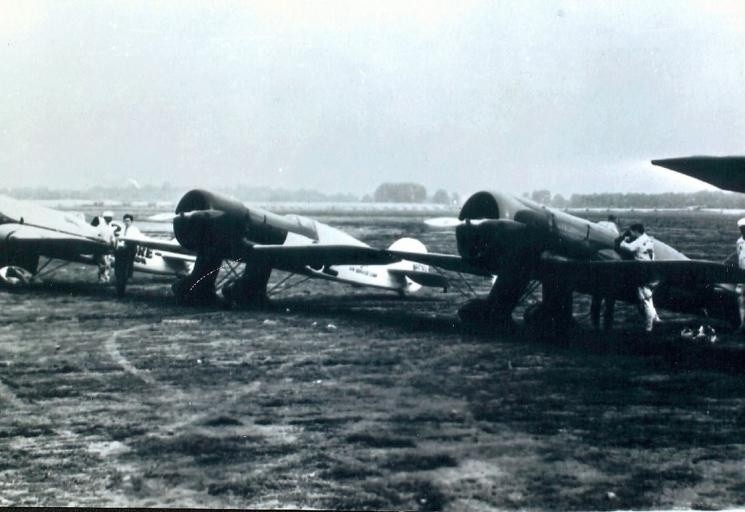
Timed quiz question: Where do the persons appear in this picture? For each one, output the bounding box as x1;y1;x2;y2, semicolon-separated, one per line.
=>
615;223;661;334
94;212;119;283
598;215;619;237
122;214;143;281
735;217;745;334
590;292;619;333
